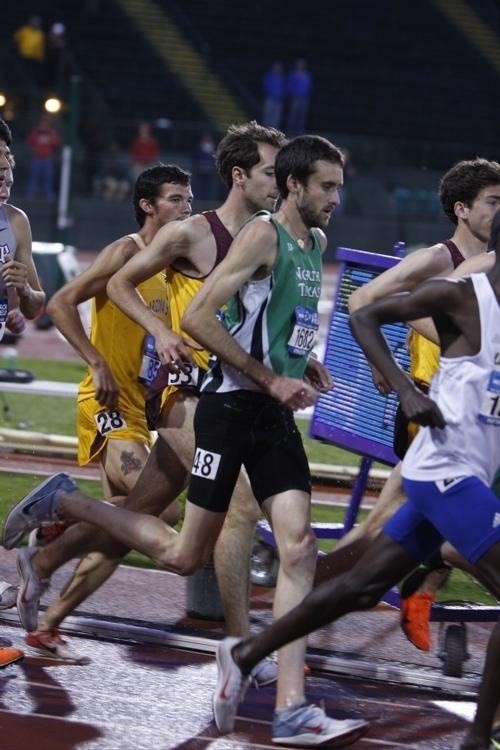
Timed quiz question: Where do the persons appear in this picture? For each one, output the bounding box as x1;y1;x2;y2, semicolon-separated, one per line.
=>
211;205;500;750
18;119;313;689
10;14;47;90
1;120;45;667
1;153;28;335
0;132;369;745
26;113;62;207
190;132;217;201
301;315;441;575
47;23;71;84
315;155;500;652
129;121;156;186
262;59;310;140
11;167;195;668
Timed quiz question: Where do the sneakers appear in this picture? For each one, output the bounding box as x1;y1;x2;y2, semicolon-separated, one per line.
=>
213;636;372;750
0;471;92;668
400;568;437;651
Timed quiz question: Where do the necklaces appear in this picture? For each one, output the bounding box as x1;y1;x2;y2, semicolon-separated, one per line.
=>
278;207;312;250
